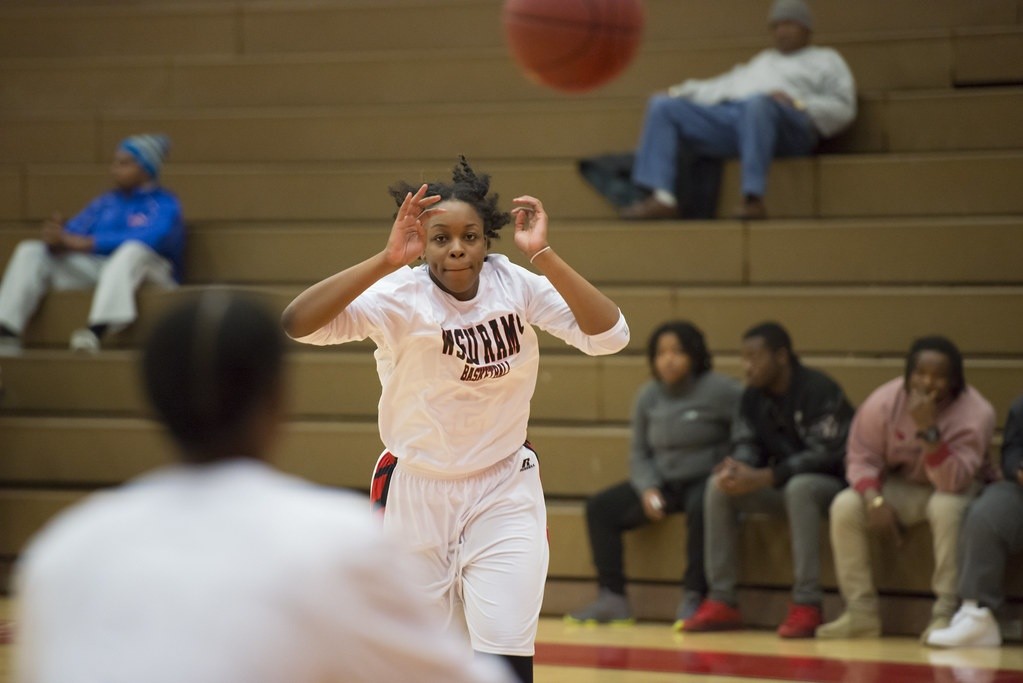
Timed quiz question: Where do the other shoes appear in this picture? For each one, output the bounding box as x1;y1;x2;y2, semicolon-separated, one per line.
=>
618;198;682;220
71;328;101;353
736;195;769;220
0;336;22;360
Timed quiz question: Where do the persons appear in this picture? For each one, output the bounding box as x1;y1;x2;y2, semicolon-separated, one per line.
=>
0;131;188;356
279;154;630;683
563;320;1023;648
623;0;858;219
12;286;512;683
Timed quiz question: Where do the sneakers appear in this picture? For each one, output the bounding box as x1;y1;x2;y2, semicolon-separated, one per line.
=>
815;604;881;639
565;588;636;630
781;607;826;639
921;605;1002;650
672;592;745;632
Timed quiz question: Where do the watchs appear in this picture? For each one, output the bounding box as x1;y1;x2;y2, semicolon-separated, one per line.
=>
918;427;940;442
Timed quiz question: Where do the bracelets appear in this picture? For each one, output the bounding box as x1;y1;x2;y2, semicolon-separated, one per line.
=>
866;496;884;508
530;246;550;264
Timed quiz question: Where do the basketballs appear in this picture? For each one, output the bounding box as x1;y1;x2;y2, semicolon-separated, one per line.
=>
504;0;643;92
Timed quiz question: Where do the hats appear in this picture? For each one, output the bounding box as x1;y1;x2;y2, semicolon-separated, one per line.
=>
767;0;816;32
122;133;170;179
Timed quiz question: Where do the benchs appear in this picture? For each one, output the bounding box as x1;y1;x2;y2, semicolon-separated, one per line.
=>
0;0;1023;593
